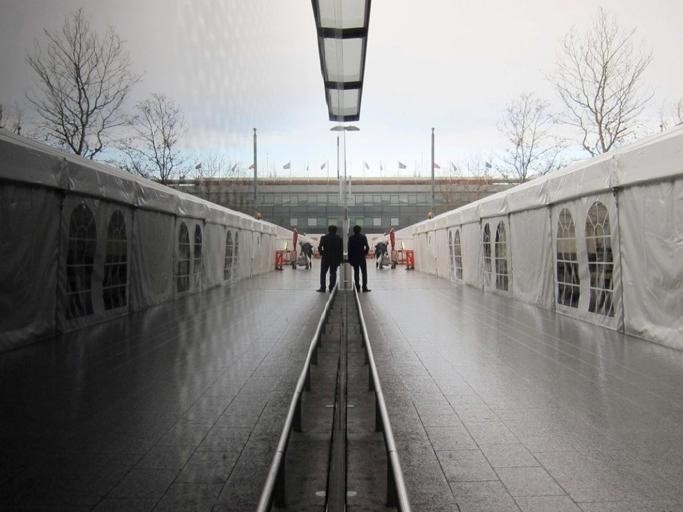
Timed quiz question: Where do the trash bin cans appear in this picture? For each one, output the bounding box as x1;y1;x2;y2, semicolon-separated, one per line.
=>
276;249;283;270
405;250;415;269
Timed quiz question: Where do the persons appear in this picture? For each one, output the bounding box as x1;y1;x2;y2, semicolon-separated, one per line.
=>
315;225;342;293
348;225;372;292
299;241;313;270
374;241;389;269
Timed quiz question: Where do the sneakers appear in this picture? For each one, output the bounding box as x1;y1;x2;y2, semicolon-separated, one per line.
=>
316;289;332;292
358;289;371;292
